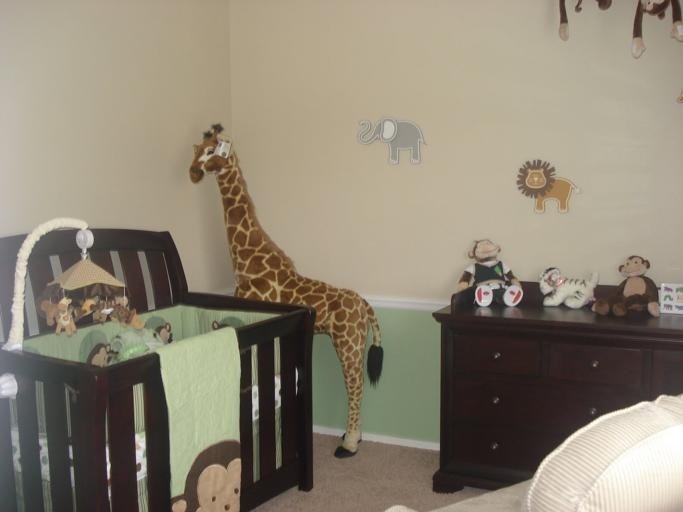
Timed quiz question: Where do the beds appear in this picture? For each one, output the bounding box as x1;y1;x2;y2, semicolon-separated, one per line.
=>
0;231;316;512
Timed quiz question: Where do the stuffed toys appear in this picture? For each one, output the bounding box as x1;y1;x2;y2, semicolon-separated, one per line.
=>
187;121;385;461
457;237;525;309
0;215;146;353
557;0;613;41
631;0;683;60
536;267;601;311
596;255;663;320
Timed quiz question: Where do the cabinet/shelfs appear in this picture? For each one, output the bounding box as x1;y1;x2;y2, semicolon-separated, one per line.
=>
431;281;683;494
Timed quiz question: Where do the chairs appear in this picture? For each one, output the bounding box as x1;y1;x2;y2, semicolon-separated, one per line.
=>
381;395;683;512
